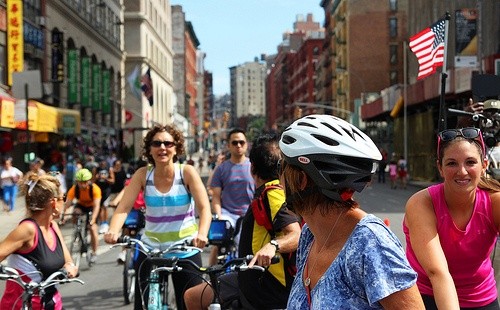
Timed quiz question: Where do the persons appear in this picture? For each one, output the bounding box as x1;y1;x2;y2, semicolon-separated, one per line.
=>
457;98;500;182
206;129;258;279
24;157;48;190
188;150;230;170
109;178;146;264
105;125;213;310
278;114;426;310
0;158;23;211
378;147;408;190
60;168;102;266
0;174;79;310
403;127;500;310
49;154;147;234
184;136;302;310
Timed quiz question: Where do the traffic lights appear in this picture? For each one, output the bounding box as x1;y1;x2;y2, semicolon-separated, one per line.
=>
295;106;303;119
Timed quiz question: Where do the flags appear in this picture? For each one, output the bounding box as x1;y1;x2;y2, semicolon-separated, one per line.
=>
143;69;154;106
127;64;142;102
409;18;445;81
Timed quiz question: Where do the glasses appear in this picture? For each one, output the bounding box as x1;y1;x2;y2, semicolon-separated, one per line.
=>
150;140;177;149
231;139;246;146
436;127;485;161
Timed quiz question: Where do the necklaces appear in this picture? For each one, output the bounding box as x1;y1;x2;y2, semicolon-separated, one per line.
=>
304;207;343;286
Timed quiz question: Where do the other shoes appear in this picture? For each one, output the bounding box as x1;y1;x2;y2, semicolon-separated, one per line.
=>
99;224;108;233
118;256;126;263
90;256;98;263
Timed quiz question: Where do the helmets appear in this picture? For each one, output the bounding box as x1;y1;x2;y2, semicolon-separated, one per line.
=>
74;168;92;182
278;114;383;204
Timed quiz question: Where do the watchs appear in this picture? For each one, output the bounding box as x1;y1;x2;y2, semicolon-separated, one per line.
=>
269;240;280;253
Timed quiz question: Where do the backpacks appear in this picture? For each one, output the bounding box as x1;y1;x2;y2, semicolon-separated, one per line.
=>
250;184;306;279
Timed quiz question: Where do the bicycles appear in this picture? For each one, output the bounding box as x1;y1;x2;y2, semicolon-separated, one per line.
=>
148;238;280;310
59;210;93;271
107;203;147;305
0;264;85;310
108;234;210;310
